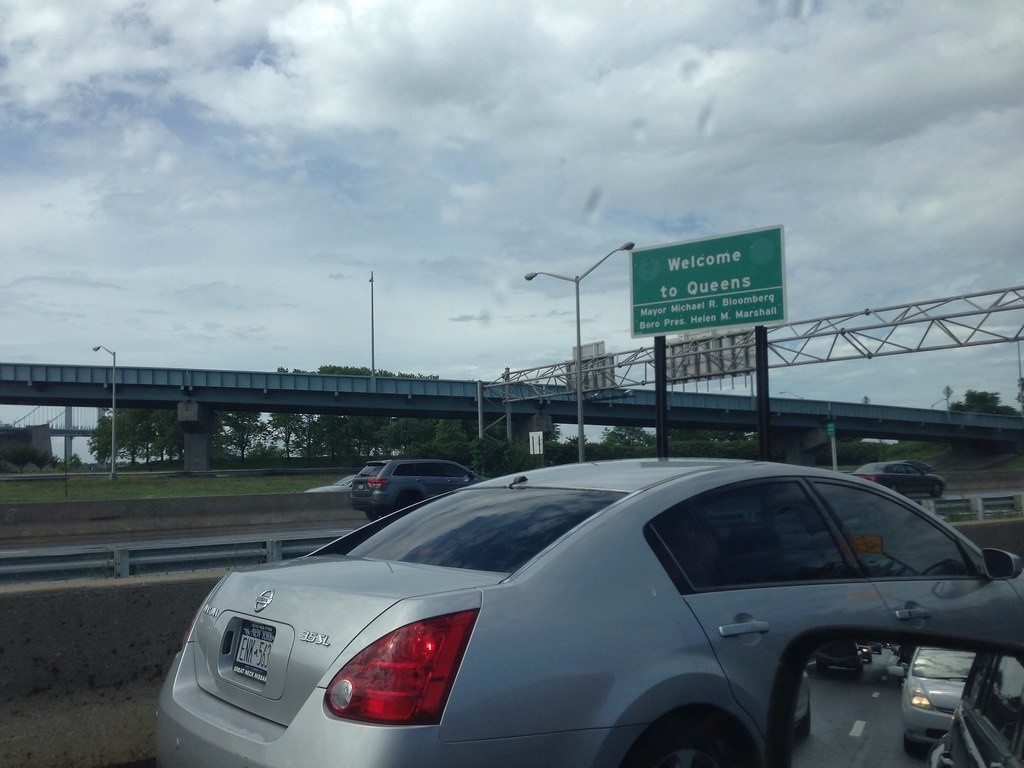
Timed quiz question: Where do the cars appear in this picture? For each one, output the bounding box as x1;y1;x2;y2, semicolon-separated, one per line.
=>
791;668;812;736
304;474;356;493
845;460;947;498
895;647;976;757
813;638;917;674
895;458;936;472
146;457;1024;768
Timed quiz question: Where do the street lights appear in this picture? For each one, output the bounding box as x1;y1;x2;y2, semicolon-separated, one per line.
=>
522;239;638;465
930;398;948;409
367;271;376;376
91;344;119;480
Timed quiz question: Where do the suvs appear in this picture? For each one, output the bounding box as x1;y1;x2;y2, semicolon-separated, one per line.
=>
349;459;492;524
